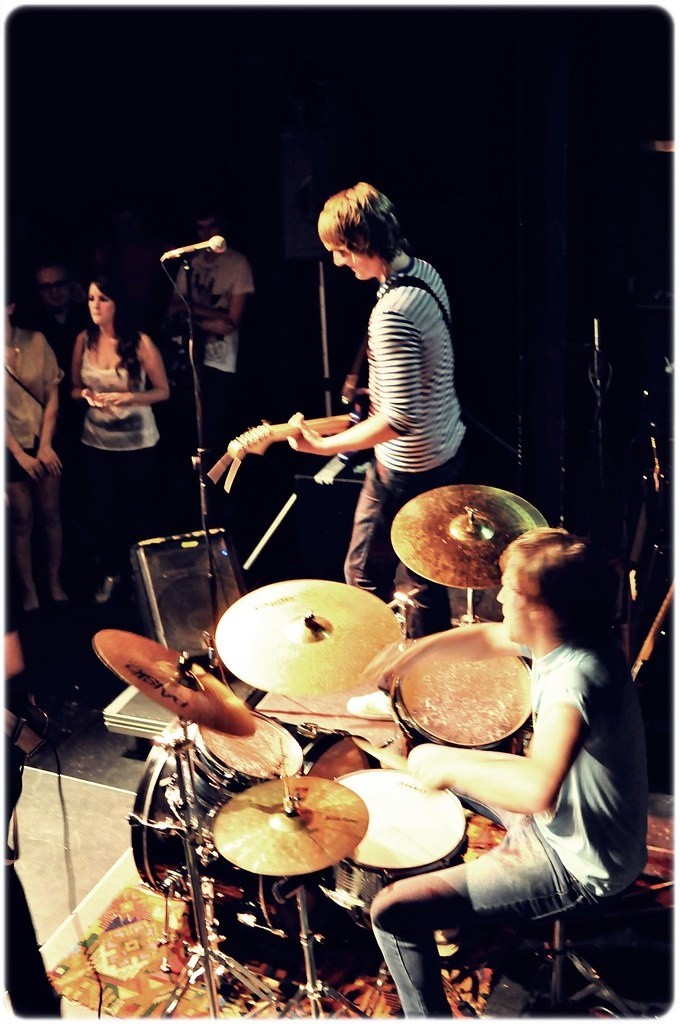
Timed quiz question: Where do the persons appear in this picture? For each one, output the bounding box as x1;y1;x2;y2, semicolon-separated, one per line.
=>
5;214;256;616
366;527;649;1016
285;181;467;605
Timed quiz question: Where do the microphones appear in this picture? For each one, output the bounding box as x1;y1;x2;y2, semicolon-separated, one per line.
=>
159;236;227;261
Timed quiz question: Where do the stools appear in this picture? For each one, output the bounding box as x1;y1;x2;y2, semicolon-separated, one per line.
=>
502;885;637;1019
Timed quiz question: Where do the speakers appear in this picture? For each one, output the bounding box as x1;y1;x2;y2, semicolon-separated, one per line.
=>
130;528;249;658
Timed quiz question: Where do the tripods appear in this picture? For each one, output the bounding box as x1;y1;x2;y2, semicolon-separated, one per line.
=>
160;713;369;1019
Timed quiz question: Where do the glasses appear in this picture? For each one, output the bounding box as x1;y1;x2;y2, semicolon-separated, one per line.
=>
38;279;68;291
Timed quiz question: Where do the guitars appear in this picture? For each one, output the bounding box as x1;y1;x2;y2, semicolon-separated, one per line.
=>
227;388;373;467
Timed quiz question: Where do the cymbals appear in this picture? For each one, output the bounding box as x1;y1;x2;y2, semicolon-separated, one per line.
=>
212;776;369;876
214;578;402;697
390;484;551;591
91;629;256;739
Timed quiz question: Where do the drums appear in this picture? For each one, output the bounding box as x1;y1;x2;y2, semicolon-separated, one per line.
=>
391;655;533;752
130;710;468;918
